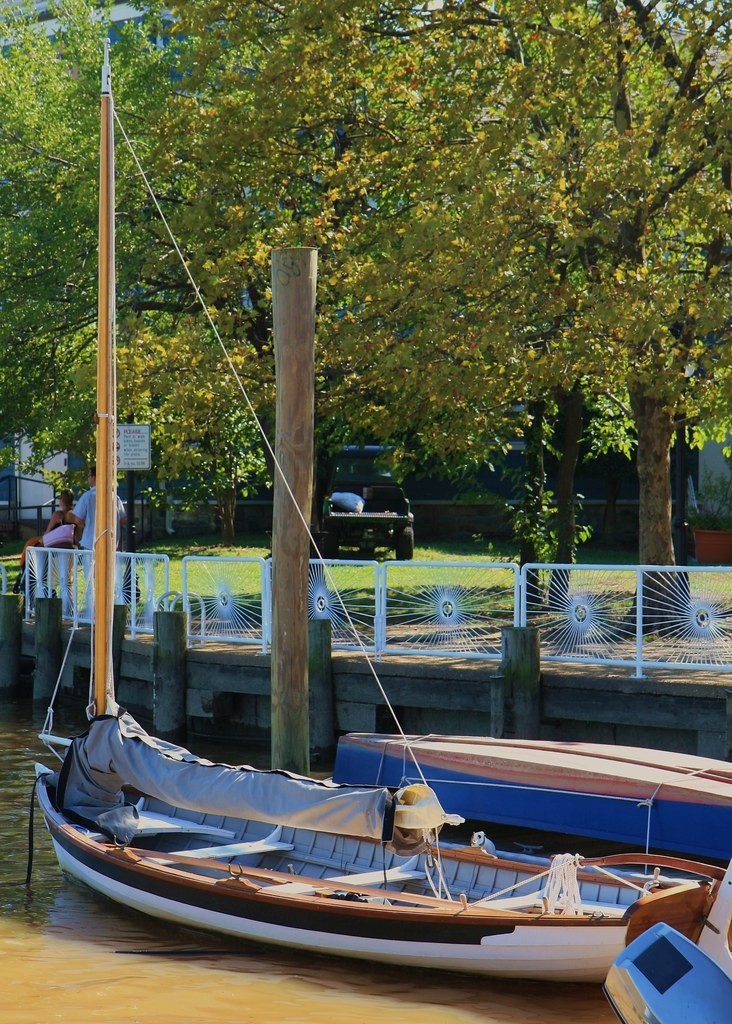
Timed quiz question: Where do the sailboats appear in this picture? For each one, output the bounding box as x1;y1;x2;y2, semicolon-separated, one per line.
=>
17;34;723;985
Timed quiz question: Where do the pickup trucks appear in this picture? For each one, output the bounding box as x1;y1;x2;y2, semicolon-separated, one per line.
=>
314;444;416;561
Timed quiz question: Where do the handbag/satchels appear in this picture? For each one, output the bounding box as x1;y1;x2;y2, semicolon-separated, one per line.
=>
42;518;75;548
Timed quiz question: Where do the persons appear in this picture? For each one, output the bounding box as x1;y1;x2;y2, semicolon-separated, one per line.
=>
72;467;127;620
45;489;85;550
